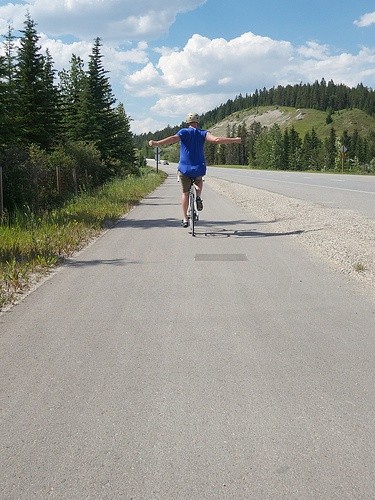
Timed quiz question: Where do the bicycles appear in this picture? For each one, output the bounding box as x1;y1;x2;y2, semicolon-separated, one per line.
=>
176;172;206;237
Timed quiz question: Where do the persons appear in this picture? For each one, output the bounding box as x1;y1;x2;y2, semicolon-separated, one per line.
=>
148;112;242;228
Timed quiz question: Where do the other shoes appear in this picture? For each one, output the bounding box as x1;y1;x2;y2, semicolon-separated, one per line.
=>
196;196;203;211
181;219;188;227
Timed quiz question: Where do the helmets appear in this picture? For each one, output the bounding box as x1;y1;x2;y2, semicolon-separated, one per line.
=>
186;112;200;124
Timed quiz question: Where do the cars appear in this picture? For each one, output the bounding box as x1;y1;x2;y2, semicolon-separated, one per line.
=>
156;159;169;165
